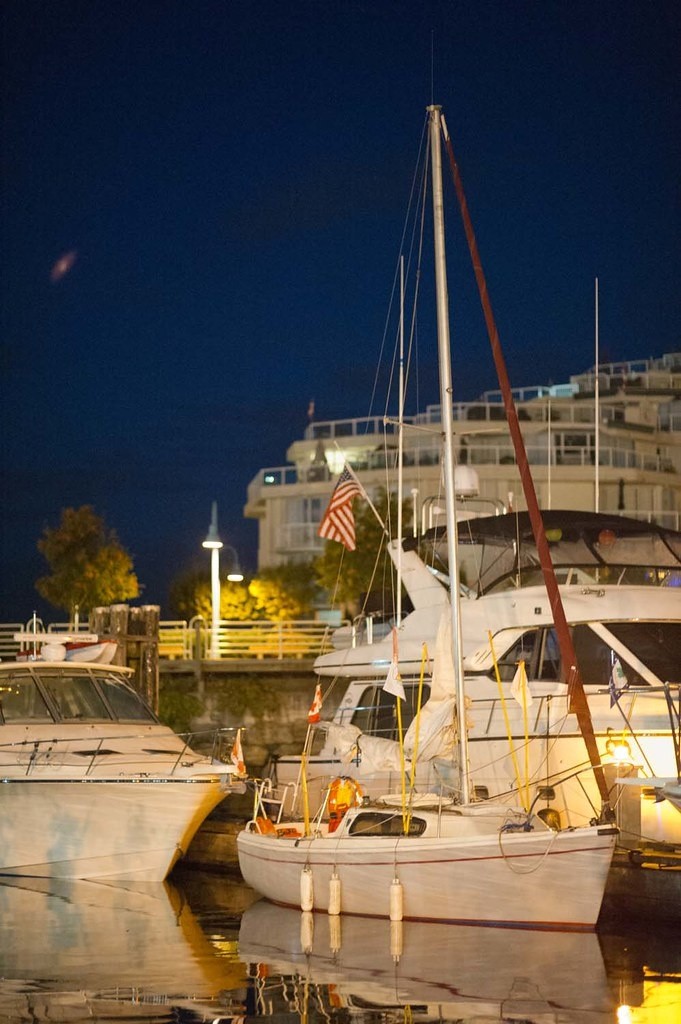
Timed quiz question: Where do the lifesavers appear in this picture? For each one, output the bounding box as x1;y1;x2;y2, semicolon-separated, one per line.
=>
326;775;365;820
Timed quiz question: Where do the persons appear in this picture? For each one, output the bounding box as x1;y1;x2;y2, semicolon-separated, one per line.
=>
327;776;366;831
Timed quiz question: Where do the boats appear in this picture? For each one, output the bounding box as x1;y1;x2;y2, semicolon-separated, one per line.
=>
0;632;240;883
258;507;681;829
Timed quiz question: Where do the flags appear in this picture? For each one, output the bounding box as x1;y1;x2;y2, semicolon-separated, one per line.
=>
387;660;407;703
308;685;323;723
511;663;534;708
230;729;246;775
319;467;367;551
610;655;629;708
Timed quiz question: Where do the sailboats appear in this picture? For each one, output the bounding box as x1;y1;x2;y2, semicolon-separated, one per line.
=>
236;101;619;931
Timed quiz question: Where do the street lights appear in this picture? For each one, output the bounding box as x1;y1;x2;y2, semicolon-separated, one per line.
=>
201;501;223;658
